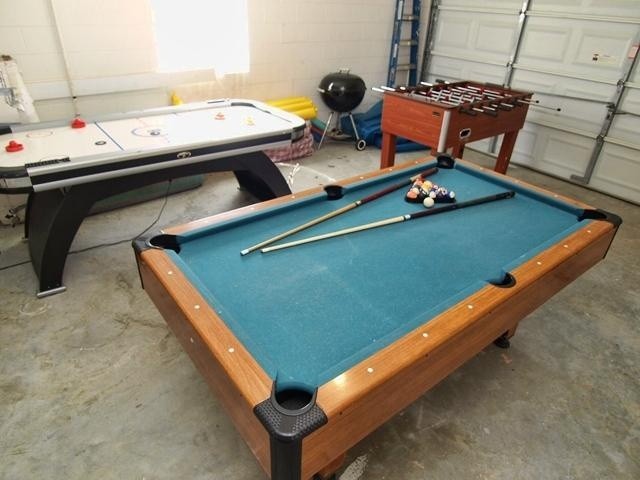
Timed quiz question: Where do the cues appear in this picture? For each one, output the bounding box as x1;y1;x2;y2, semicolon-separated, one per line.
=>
260;190;515;252
238;166;439;256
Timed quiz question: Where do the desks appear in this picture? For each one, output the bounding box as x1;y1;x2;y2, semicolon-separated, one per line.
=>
372;78;534;176
1;99;307;300
134;152;621;476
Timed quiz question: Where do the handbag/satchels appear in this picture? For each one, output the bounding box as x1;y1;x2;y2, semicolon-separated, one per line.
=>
262;121;314;164
